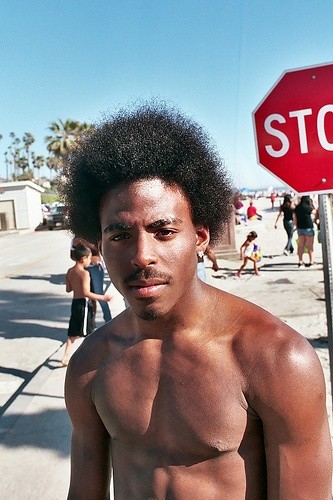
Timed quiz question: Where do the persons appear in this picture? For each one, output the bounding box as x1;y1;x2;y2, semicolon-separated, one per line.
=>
275;194;296;256
270;193;275;210
72;236;112;336
314;208;320;229
65;109;333;500
246;201;262;220
62;243;112;366
294;195;315;267
197;248;218;282
237;231;260;277
234;192;248;226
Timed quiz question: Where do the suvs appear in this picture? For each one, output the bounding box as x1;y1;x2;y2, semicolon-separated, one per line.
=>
46;206;71;230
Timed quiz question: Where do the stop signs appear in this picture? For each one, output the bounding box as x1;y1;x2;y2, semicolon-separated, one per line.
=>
251;61;333;195
